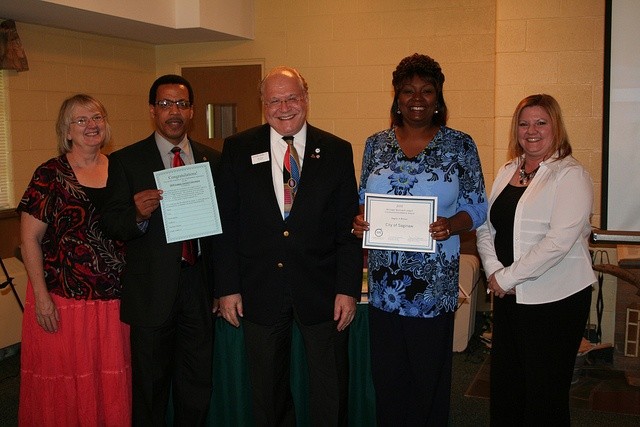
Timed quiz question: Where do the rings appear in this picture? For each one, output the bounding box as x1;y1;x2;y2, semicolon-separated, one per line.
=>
351;229;355;234
446;228;451;236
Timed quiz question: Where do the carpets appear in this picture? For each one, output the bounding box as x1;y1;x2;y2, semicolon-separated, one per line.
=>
463;344;639;416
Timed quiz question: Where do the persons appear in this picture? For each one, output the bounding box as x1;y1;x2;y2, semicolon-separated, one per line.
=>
349;52;487;427
475;96;599;427
16;93;135;427
213;65;359;427
101;73;223;426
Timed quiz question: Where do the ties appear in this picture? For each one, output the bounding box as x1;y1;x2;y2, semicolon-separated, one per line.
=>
282;136;301;220
171;147;198;267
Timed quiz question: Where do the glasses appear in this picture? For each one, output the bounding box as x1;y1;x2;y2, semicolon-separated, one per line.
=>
264;98;306;106
69;115;104;127
156;100;191;109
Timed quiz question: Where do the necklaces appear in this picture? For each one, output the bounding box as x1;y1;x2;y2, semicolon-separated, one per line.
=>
519;160;541;185
